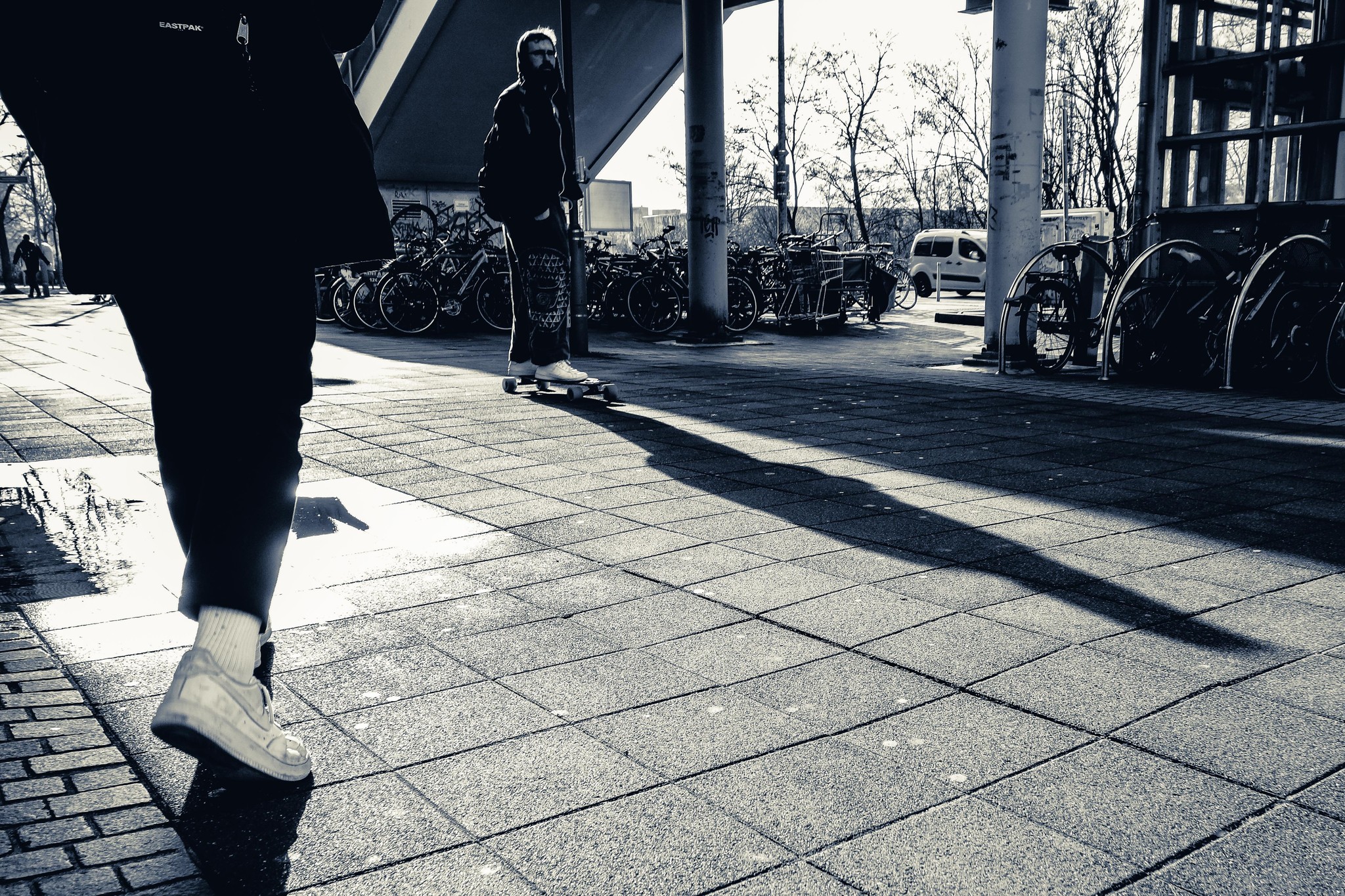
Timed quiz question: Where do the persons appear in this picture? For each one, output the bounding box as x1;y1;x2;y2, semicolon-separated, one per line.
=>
0;0;378;784
13;234;52;297
38;237;54;294
486;31;590;383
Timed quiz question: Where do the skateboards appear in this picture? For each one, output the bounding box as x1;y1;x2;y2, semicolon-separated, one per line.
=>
501;375;621;401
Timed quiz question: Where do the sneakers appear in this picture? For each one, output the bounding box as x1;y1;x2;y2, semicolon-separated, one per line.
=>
508;360;536;376
535;360;588;381
150;648;312;781
255;612;271;668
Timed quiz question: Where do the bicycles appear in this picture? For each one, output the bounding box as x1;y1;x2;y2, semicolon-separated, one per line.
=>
1004;210;1345;401
314;196;918;339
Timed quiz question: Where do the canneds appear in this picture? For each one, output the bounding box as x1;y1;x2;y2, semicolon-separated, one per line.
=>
575;156;586;182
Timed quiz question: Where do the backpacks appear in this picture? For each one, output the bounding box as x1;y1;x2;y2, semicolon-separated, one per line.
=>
478;126;505;223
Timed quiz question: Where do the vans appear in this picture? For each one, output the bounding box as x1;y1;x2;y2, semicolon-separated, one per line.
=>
908;229;987;298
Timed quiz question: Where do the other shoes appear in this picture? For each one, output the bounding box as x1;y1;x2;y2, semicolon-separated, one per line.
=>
89;295;101;302
27;293;34;297
35;294;42;298
